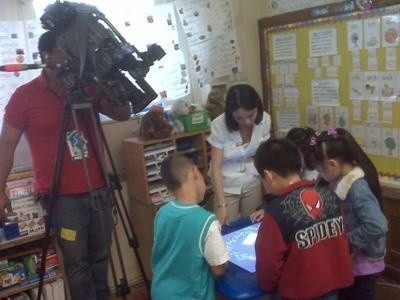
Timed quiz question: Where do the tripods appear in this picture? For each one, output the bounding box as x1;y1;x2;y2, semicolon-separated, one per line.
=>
36;89;150;300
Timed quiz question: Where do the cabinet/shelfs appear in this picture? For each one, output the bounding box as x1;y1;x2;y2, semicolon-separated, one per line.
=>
123;127;213;282
0;169;72;300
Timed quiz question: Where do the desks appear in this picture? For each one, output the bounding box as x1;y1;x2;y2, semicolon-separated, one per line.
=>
214;215;273;300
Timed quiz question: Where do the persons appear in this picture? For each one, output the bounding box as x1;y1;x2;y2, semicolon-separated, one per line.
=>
249;127;388;300
0;31;131;300
207;84;272;226
150;156;229;300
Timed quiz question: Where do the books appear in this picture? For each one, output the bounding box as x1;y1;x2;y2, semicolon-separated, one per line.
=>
0;179;66;300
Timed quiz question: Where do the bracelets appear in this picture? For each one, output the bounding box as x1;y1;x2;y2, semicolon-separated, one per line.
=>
216;203;227;208
